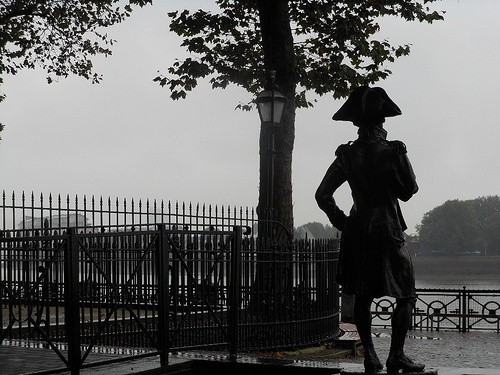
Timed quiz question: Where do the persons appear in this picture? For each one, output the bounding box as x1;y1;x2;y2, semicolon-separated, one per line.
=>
314;87;426;374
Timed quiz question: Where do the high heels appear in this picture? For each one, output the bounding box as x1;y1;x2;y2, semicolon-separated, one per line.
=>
362;360;384;374
387;357;425;373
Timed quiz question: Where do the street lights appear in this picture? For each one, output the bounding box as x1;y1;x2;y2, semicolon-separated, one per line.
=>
255;68;287;310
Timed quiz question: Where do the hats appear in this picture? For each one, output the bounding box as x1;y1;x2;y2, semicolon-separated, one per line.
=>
332;87;402;120
